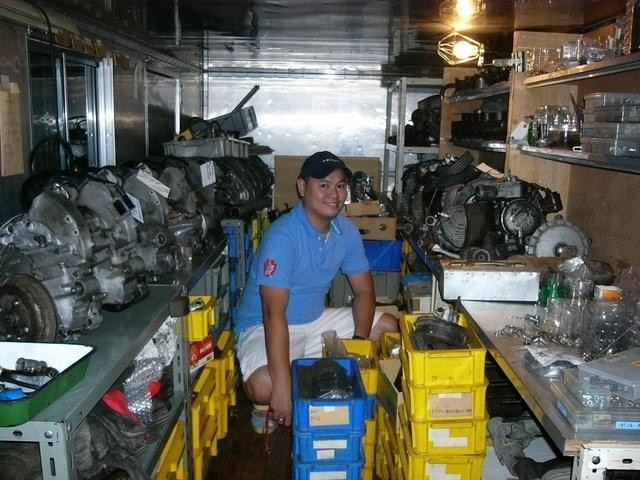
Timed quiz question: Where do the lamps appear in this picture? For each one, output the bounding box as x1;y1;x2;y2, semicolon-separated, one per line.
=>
437;34;483;64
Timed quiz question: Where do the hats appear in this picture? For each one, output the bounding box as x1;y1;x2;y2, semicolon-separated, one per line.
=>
298;150;354;183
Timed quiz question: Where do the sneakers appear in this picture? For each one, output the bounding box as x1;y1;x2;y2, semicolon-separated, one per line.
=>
250;403;280;435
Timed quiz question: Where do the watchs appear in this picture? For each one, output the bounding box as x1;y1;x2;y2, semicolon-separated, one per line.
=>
353;335;369;340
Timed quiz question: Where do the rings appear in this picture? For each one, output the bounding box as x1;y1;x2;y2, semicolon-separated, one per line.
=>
279;417;285;421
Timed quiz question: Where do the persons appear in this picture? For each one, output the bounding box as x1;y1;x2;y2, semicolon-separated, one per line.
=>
235;152;399;434
